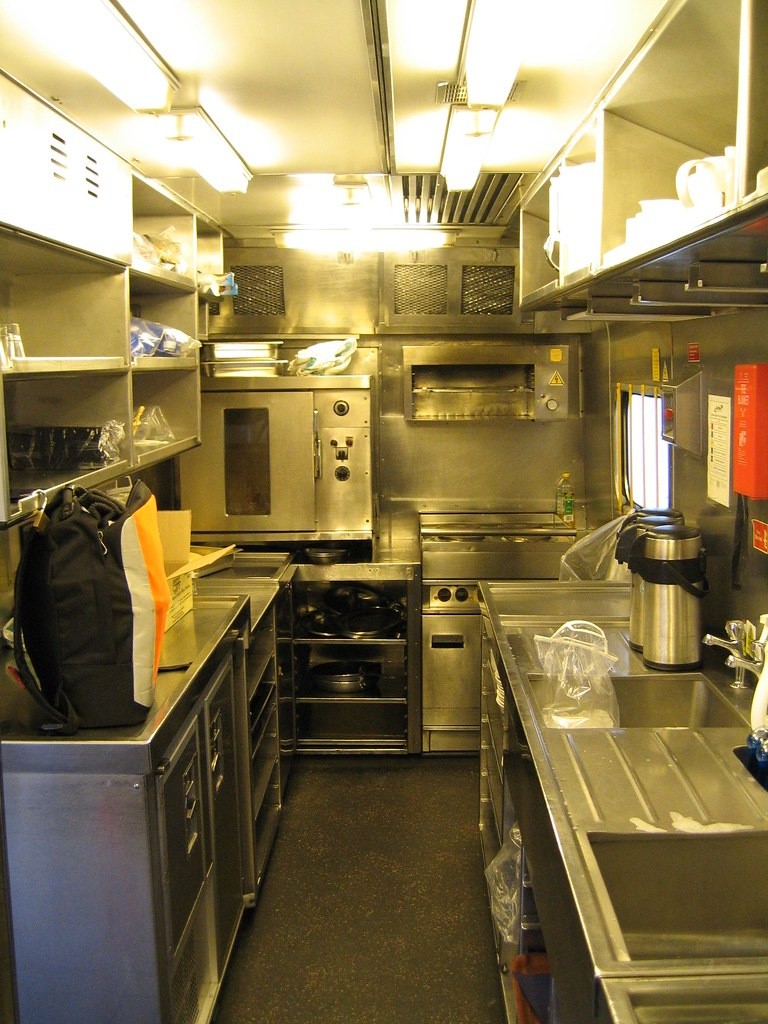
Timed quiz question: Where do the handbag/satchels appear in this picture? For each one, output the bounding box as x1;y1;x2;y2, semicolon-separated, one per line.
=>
7;478;172;735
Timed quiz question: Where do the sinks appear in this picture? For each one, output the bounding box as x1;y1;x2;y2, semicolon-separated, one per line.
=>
526;672;749;729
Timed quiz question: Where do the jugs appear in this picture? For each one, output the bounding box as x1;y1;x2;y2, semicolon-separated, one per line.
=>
615;507;711;672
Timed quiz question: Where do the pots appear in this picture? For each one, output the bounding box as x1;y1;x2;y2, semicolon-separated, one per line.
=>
310;660;382;692
303;546;353;563
295;584;398;639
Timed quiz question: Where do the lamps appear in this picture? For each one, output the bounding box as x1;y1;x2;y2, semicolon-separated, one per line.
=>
1;0;549;194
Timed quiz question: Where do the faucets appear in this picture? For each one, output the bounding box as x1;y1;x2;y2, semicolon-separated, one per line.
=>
726;641;765;678
701;619;749;690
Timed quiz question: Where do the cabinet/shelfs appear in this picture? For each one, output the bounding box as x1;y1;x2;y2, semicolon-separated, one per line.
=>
479;616;559;1024
156;540;421;1024
1;69;224;531
520;0;767;316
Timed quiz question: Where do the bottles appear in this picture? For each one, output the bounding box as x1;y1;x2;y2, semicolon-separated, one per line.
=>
556;472;575;526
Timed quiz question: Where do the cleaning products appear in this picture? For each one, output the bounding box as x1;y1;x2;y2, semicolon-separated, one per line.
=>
750;612;767;734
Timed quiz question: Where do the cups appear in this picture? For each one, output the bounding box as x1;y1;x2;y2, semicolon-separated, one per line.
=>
0;323;25;356
